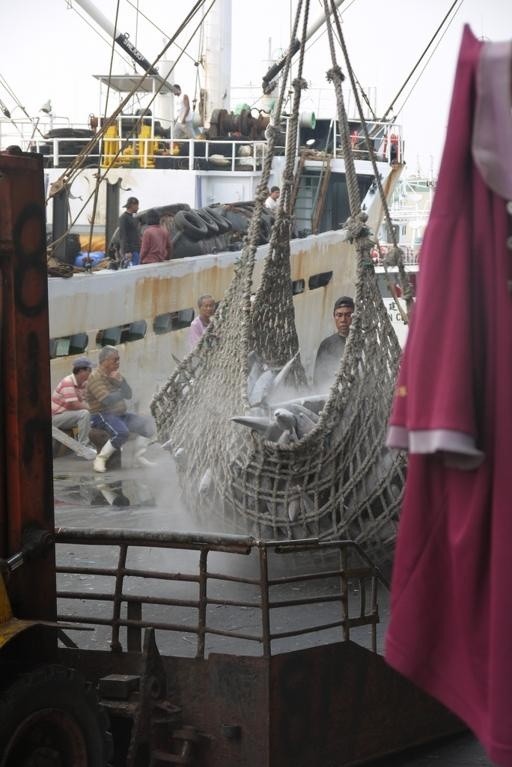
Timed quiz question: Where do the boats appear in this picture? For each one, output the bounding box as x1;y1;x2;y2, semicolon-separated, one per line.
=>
363;141;447;352
0;0;416;506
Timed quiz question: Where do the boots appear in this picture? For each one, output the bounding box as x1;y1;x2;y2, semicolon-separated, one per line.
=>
93;441;117;473
130;434;157;469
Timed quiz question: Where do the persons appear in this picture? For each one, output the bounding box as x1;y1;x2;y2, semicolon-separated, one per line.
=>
162;84;196;155
87;344;154;473
313;296;354;393
264;186;286;211
51;357;97;456
119;197;141;268
139;208;172;263
191;295;216;347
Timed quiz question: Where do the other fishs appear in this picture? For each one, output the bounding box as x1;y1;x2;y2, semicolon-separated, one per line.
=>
146;349;409;577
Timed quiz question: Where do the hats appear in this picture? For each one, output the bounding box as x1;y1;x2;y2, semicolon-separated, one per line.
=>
122;196;140;208
333;296;355;309
72;357;99;369
